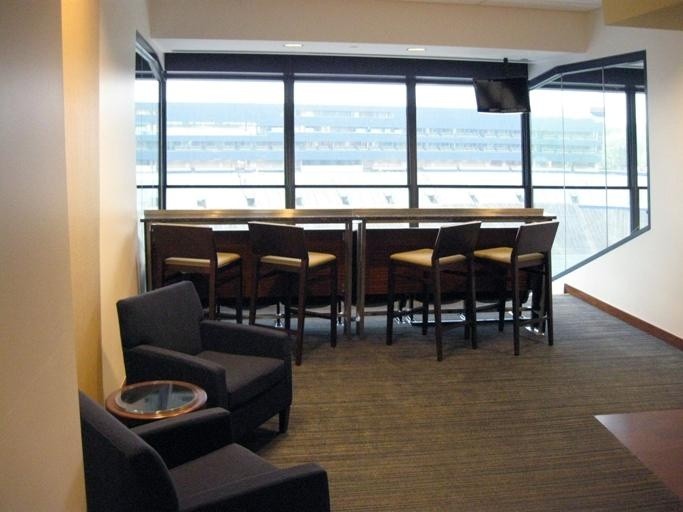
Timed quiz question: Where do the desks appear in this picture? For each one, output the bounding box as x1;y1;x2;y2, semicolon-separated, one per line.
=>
103;378;209;422
138;217;358;342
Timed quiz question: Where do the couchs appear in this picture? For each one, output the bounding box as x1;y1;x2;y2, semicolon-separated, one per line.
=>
116;279;297;444
77;386;329;512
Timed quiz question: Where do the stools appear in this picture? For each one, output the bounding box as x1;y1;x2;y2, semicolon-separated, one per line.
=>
384;220;484;361
473;218;561;356
152;222;245;326
247;219;340;370
